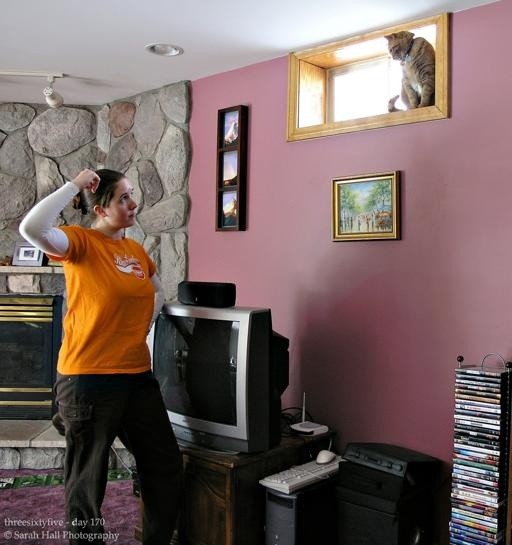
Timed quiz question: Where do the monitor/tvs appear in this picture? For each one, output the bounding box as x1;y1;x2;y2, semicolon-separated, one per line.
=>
146;302;289;458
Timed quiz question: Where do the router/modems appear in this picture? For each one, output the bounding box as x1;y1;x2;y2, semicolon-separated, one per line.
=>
289;392;329;436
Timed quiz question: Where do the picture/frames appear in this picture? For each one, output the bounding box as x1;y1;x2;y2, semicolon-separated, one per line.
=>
214;104;250;231
12;242;46;267
331;169;402;242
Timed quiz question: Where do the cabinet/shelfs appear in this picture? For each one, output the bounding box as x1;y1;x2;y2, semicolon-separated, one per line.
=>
131;413;338;545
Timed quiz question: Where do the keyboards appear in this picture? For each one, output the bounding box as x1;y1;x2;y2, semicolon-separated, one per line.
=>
258;453;348;495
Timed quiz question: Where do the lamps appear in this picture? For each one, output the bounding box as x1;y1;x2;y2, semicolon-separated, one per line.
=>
42;75;64;109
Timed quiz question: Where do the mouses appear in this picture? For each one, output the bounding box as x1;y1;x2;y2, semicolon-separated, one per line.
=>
315;450;336;464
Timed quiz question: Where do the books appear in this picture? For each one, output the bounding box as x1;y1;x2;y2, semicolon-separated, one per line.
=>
448;363;511;545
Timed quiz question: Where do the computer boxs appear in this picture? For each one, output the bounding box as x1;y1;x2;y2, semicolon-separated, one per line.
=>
265;476;337;545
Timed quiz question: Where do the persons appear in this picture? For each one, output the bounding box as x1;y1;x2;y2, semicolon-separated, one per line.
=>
17;167;189;545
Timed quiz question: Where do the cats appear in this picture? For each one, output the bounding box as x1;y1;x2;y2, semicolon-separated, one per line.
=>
384;31;435;112
